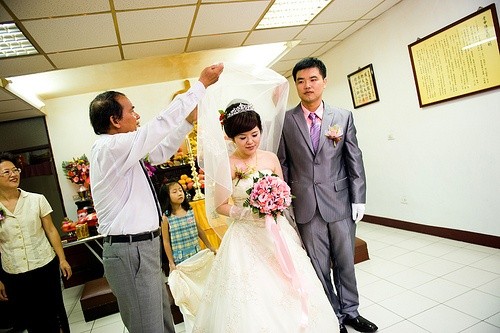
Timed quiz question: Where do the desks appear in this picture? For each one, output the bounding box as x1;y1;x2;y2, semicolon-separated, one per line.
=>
189;199;228;256
63;234;103;264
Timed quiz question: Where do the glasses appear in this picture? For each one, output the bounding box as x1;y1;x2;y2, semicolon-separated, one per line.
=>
0;168;21;176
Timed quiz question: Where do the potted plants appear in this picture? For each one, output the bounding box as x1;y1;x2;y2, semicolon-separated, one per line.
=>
62;154;96;216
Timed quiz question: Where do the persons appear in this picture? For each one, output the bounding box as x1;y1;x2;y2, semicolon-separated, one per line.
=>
161;180;217;273
89;61;225;333
0;153;72;333
169;98;340;333
261;56;378;333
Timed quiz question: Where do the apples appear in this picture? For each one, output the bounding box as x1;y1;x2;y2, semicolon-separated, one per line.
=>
177;174;194;190
197;169;205;183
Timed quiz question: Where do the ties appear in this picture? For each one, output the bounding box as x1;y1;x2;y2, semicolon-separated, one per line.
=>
309;113;320;153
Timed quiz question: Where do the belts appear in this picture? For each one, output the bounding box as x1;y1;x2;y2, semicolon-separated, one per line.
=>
104;227;161;243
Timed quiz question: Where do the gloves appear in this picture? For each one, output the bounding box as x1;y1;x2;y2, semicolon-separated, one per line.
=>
229;206;264;221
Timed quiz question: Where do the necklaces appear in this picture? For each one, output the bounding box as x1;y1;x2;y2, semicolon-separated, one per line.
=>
239;153;258;168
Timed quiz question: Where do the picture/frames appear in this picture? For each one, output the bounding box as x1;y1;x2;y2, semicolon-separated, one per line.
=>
347;63;380;109
408;3;500;108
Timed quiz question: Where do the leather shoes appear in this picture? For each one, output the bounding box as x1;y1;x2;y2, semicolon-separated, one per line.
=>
340;324;348;333
342;315;378;333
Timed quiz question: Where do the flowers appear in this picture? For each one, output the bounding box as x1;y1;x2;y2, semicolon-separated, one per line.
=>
143;152;156;177
324;124;343;147
234;164;296;225
